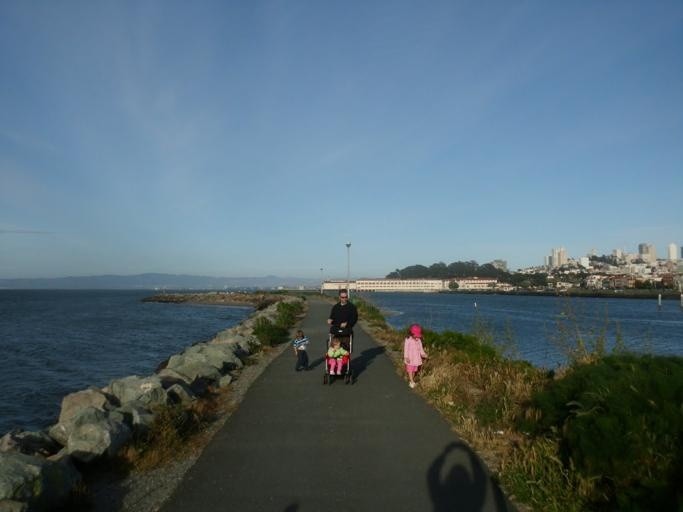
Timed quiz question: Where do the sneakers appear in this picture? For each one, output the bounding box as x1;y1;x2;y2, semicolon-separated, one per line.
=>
295;366;311;372
329;371;342;375
409;382;416;388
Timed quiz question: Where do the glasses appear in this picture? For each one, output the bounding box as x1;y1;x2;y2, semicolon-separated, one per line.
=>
340;296;348;299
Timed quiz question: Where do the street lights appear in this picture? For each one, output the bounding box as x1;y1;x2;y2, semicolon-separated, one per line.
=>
320;268;324;282
345;241;351;298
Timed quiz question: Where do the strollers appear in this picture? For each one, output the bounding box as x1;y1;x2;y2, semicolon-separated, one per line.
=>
322;325;356;386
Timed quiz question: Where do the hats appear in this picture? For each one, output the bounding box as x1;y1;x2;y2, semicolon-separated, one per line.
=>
409;324;422;338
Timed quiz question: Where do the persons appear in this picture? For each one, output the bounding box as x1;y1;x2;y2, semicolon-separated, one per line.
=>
326;335;349;375
402;324;429;388
293;330;312;370
327;289;358;334
